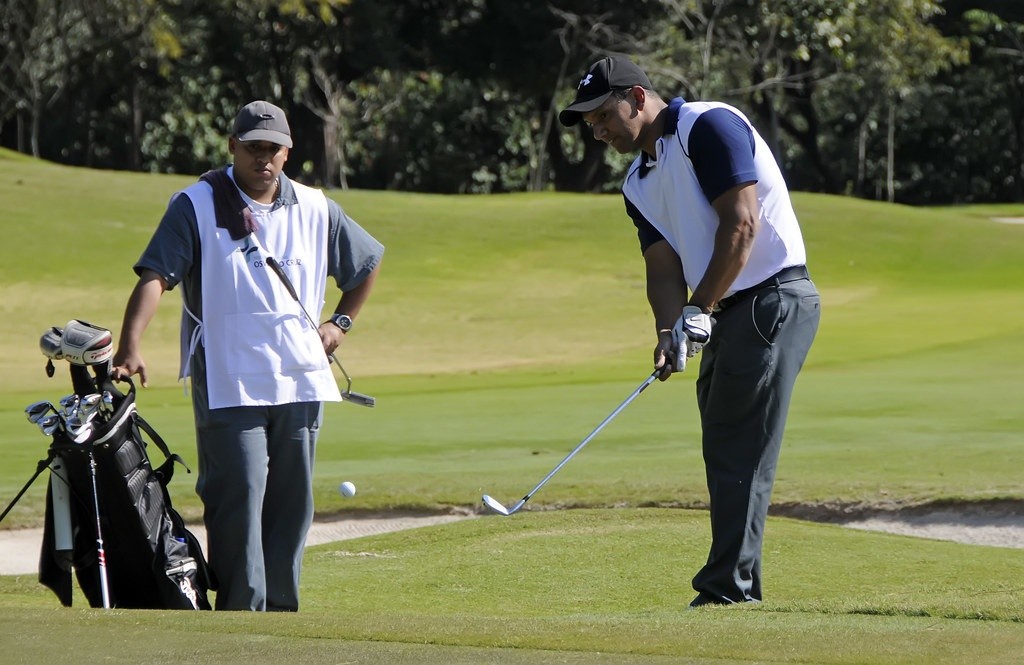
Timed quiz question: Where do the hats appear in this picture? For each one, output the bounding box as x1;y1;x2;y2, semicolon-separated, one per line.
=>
232;102;293;148
559;56;652;127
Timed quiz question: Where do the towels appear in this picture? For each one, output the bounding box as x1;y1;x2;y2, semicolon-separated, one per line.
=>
198;166;260;241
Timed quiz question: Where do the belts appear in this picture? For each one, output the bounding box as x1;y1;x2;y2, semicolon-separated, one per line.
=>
713;265;809;312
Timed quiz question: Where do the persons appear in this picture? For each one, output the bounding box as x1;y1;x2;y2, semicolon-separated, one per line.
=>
581;54;822;604
103;100;387;611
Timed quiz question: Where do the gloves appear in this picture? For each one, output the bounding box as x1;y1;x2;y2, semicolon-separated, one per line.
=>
672;306;712;372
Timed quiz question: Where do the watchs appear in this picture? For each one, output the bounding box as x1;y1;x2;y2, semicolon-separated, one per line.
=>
329;313;353;334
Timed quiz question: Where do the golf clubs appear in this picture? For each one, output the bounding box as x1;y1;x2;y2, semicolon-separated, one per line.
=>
482;369;664;517
24;391;114;446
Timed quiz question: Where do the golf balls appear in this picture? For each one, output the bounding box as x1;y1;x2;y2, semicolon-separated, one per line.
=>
340;481;357;498
